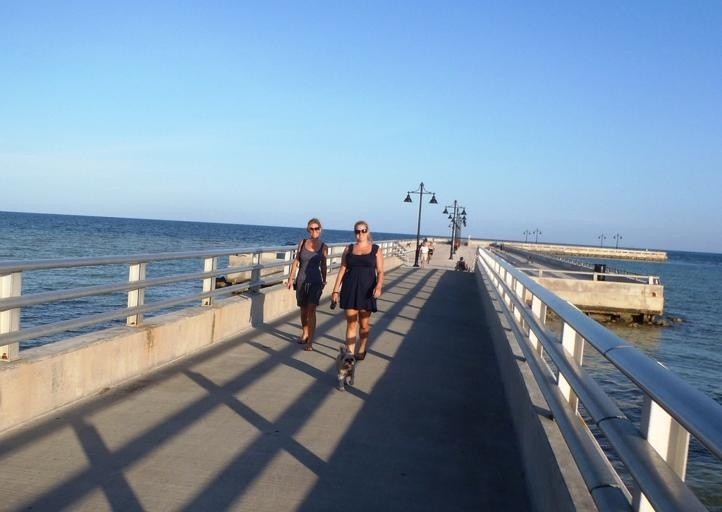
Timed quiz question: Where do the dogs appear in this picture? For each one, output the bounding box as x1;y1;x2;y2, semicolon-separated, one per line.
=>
335;345;357;391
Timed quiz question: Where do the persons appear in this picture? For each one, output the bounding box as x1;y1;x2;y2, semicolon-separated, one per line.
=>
288;218;328;351
419;242;428;268
331;221;384;360
455;257;467;271
428;242;435;264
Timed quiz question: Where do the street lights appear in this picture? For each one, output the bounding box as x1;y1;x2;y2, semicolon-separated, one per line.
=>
533;228;542;244
597;232;606;246
403;181;439;267
613;232;623;248
443;200;467;260
523;229;531;242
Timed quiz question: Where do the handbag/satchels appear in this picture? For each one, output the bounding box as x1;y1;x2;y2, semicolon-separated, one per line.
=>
292;281;297;291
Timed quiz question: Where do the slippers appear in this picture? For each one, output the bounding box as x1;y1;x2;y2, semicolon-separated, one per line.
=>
356;351;366;361
298;336;313;351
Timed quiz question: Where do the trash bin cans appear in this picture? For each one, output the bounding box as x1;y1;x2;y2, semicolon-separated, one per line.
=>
593;264;606;280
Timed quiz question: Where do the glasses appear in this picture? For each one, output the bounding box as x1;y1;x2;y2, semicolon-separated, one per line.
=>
354;230;368;234
310;228;320;231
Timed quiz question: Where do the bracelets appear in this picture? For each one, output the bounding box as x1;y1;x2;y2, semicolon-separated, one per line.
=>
333;292;338;293
